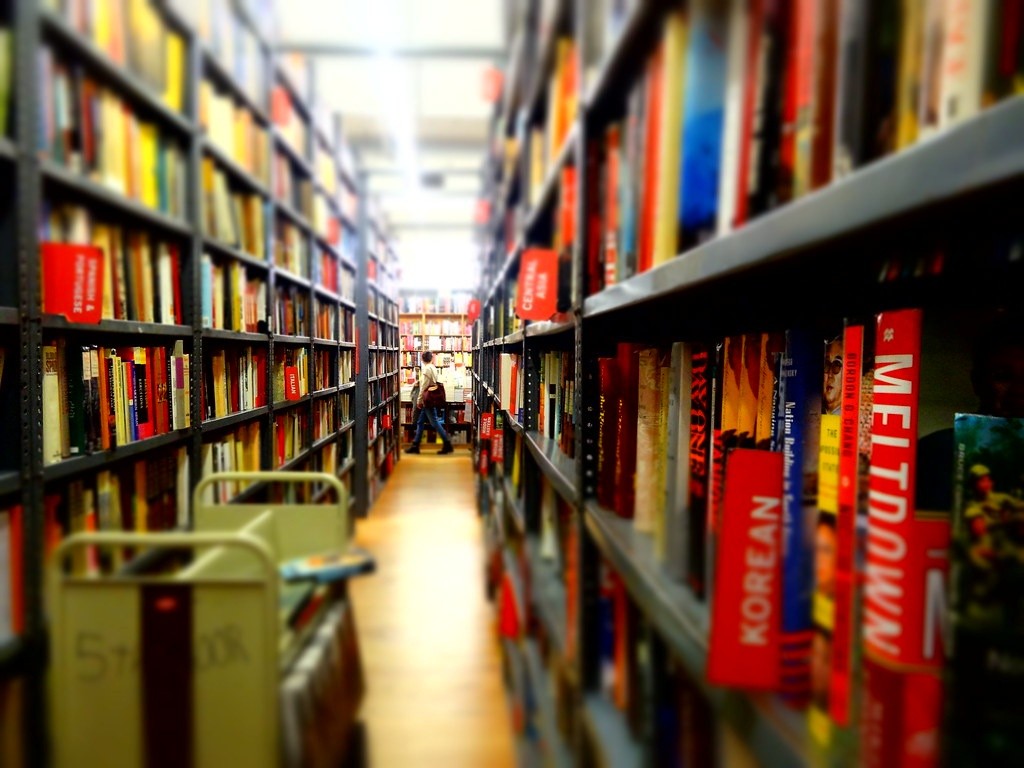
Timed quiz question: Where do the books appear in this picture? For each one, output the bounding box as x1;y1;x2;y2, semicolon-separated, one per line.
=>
471;0;1024;768
0;0;398;768
399;290;473;445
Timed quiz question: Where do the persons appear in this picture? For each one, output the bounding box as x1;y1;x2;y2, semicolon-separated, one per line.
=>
404;351;454;455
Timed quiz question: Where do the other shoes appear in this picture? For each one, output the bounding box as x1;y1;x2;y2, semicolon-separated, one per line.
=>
436;446;452;455
405;446;419;453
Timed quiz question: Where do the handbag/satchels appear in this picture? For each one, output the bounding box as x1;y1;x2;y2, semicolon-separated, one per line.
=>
422;366;445;408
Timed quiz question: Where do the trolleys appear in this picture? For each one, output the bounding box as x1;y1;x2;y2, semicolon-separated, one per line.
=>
43;473;370;768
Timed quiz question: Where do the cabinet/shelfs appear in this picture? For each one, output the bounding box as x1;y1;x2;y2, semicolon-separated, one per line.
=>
465;0;1023;768
398;312;472;448
1;0;401;768
